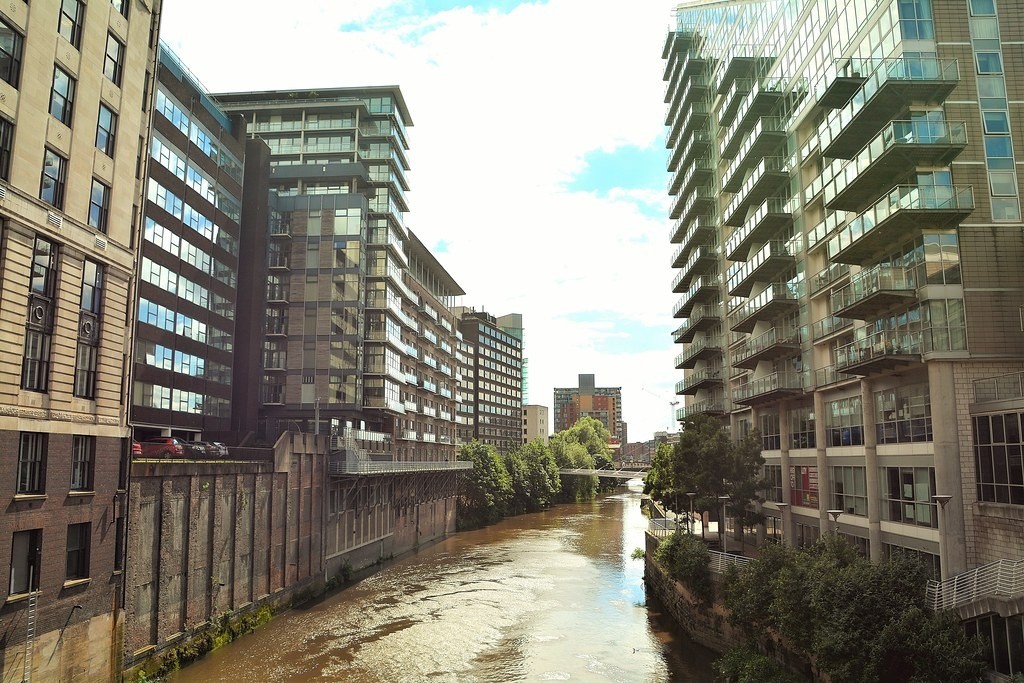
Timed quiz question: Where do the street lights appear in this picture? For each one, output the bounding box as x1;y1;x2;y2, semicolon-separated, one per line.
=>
826;508;845;547
774;502;791;564
686;492;697;542
931;494;955;607
718;496;731;574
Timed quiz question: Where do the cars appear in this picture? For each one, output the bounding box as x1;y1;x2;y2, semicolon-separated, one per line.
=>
133;439;142;460
138;436;230;460
